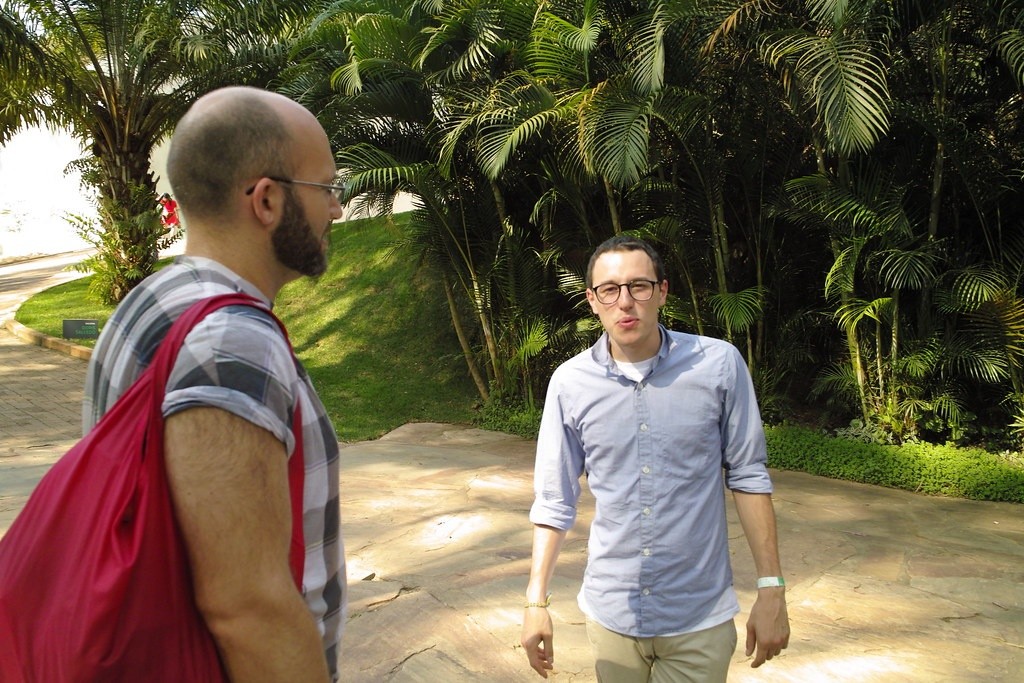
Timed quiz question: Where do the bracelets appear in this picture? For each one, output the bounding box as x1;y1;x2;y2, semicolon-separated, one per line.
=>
524;592;552;607
757;576;786;589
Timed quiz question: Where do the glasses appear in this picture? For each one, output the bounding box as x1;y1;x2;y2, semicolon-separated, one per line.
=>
592;279;661;304
246;176;346;205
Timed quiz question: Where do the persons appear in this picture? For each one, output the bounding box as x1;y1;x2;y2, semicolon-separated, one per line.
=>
80;87;347;683
160;193;181;240
521;235;790;683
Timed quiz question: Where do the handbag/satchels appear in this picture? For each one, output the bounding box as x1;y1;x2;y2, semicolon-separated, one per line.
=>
0;294;306;683
